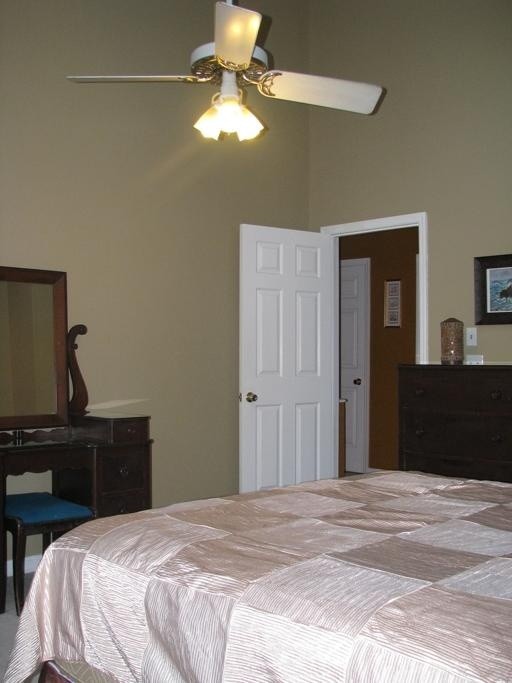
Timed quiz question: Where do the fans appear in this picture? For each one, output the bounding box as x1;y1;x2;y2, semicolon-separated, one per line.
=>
66;0;384;115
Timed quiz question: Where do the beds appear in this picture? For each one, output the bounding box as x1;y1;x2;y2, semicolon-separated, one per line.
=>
46;470;512;683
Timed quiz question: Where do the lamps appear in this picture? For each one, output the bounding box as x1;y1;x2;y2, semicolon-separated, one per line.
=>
192;72;264;141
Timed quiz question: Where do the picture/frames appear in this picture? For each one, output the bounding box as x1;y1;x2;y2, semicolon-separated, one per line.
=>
474;254;512;325
384;279;403;329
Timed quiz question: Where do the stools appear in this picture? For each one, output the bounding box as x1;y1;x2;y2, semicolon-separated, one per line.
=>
6;492;95;617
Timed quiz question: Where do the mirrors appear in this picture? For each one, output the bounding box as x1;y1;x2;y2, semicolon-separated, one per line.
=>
0;266;69;431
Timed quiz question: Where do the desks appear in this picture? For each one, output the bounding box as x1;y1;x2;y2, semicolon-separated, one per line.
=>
0;409;154;614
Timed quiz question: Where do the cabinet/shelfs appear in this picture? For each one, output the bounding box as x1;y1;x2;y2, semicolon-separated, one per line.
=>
396;364;512;484
